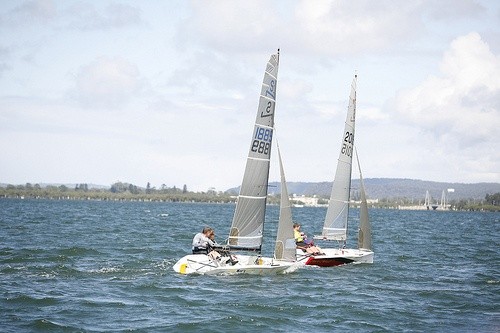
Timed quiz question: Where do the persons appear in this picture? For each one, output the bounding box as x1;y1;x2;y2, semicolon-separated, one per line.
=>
207;233;217;244
293;223;323;255
192;227;239;266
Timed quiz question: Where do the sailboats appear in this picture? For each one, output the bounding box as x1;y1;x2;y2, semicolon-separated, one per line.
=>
173;47;300;278
294;71;375;267
